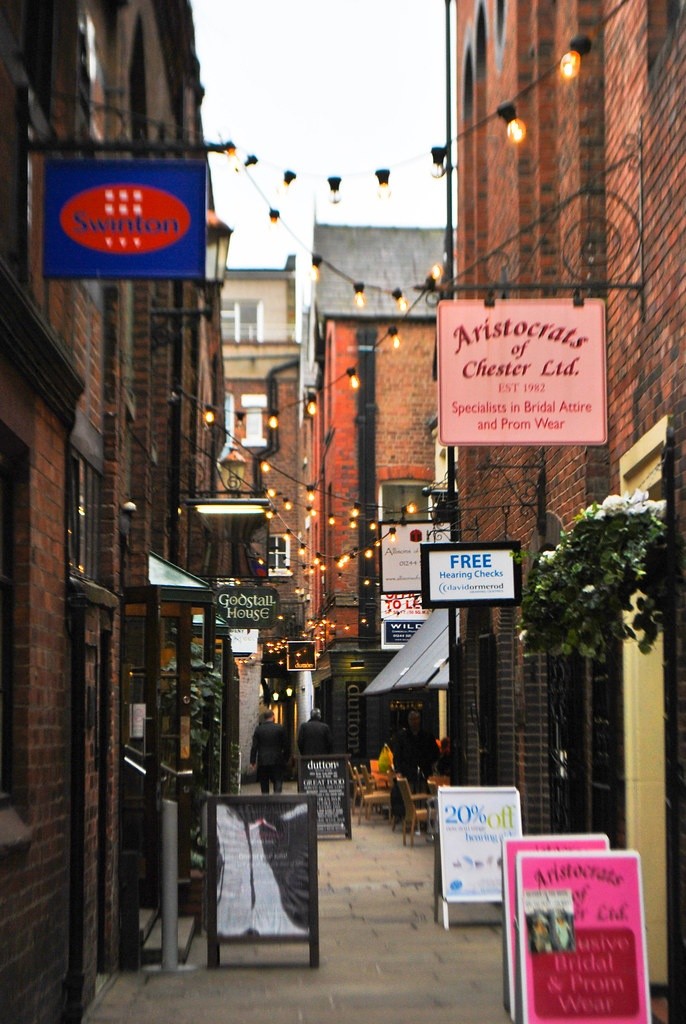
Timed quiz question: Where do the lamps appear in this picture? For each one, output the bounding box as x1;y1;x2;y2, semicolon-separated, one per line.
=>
180;442;269;499
272;691;280;700
286;686;294;697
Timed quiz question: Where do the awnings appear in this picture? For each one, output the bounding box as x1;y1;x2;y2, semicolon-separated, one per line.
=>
362;608;459;695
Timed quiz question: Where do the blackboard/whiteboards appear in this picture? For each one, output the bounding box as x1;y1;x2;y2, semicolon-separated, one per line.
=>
296;753;354;835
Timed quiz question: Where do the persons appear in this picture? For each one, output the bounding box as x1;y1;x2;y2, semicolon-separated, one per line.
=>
297;709;332;757
391;713;450;830
250;711;290;795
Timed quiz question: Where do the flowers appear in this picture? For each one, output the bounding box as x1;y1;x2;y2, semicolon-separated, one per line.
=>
509;488;670;663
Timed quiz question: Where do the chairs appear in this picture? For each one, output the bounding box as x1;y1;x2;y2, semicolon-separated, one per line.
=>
396;774;437;848
354;767;392;827
347;762;378;815
360;765;390;820
